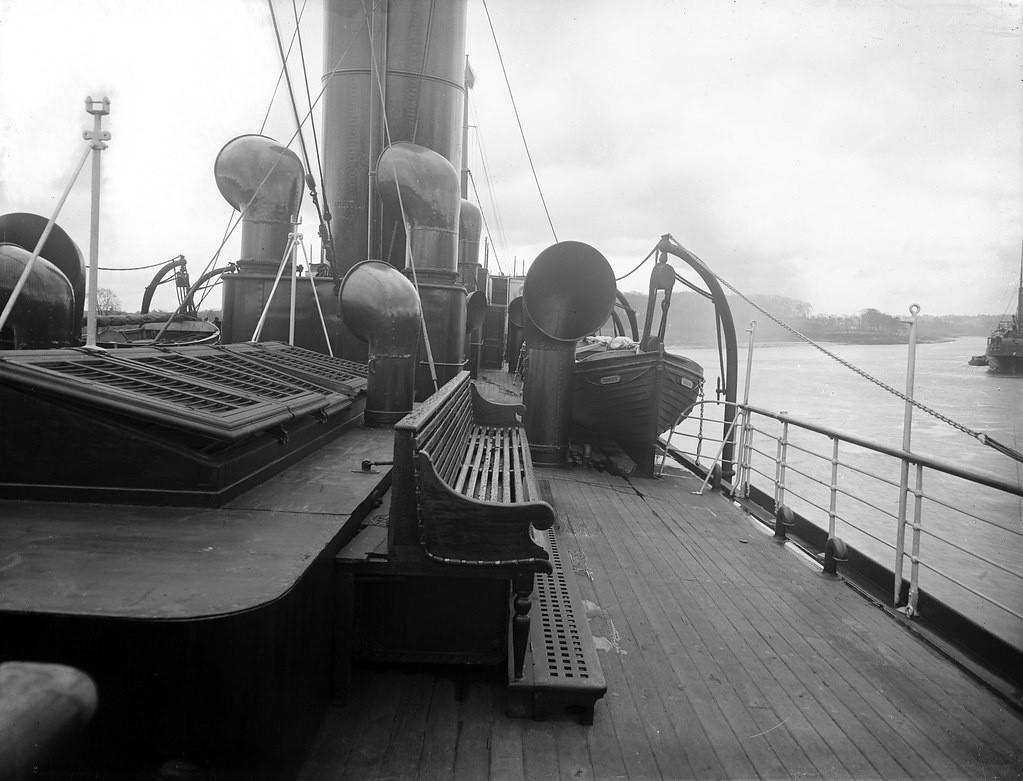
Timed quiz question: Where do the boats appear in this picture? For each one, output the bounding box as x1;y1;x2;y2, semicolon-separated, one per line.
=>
573;334;706;450
973;240;1023;377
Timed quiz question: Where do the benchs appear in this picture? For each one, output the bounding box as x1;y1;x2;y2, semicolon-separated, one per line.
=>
388;368;588;674
